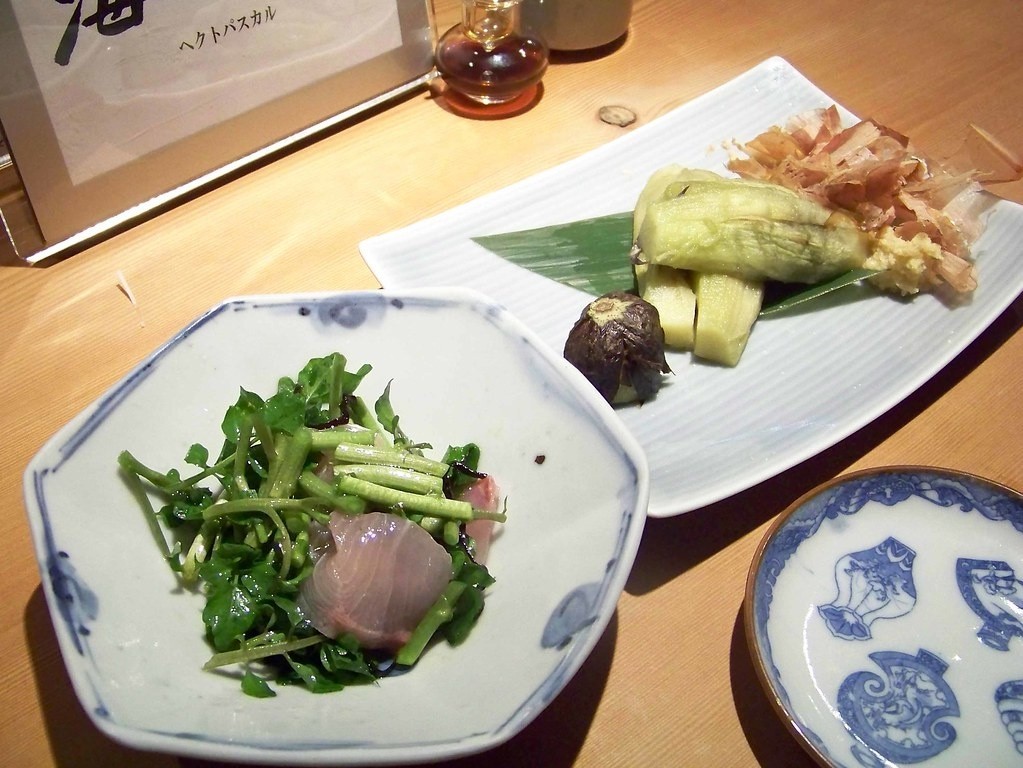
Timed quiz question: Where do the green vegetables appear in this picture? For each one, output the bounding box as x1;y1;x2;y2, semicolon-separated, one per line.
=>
114;351;509;700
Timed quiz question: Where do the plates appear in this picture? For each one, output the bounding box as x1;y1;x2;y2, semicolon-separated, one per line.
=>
360;55;1023;518
744;465;1023;768
21;294;649;765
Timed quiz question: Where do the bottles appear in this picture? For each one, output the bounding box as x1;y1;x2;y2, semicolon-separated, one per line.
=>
437;0;550;121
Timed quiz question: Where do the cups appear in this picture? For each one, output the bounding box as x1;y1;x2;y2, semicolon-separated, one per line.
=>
519;0;632;51
